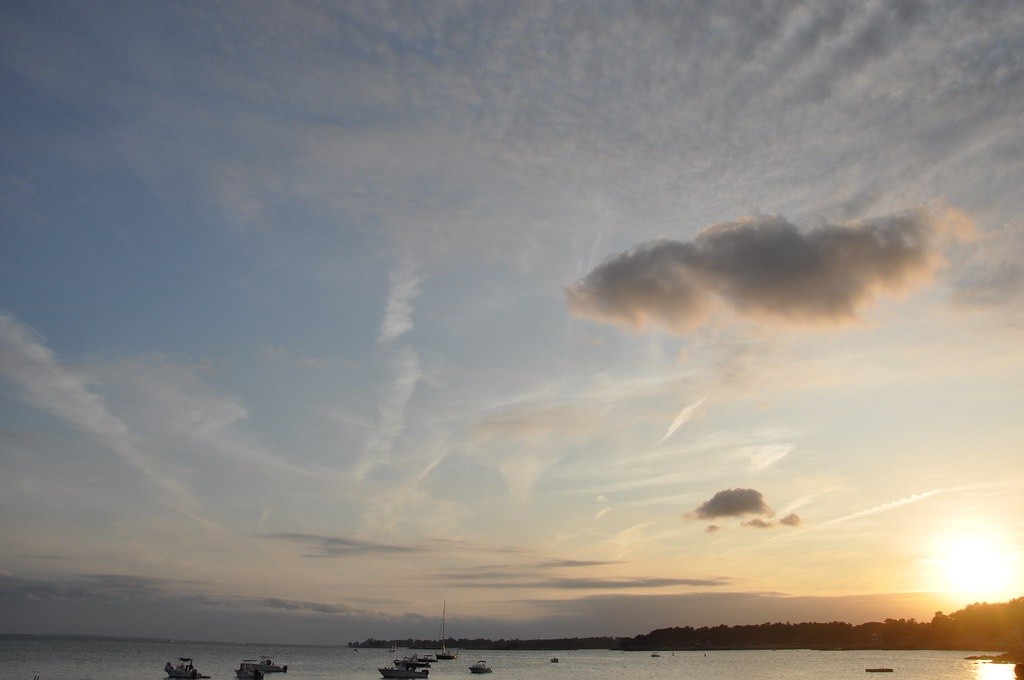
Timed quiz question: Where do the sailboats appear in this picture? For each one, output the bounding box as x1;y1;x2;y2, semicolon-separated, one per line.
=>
387;641;398;653
435;599;458;659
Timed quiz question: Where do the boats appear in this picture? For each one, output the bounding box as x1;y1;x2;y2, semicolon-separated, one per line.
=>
651;652;660;657
551;658;559;663
235;668;265;680
866;667;893;672
468;660;493;674
377;668;429;680
243;655;288;673
165;658;210;680
391;656;438;667
